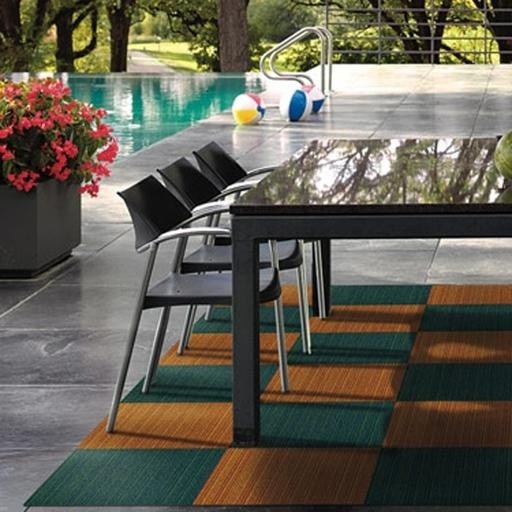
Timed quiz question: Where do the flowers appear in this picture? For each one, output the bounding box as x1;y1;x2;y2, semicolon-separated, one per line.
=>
1;77;118;198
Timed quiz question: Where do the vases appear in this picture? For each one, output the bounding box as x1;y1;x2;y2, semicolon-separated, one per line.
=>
0;177;82;280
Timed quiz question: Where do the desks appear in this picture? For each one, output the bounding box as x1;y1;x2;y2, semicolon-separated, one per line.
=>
232;137;511;449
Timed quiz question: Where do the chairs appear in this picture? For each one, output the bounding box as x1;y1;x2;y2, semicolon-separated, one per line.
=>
105;174;288;435
191;142;325;344
157;157;313;356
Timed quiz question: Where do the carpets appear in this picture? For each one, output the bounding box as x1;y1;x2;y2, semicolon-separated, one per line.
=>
23;283;512;506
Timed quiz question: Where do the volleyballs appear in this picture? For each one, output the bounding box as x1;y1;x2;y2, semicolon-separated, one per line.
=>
278;86;326;122
232;93;266;125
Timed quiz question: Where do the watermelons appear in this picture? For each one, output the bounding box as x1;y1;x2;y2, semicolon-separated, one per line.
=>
495;184;512;203
494;130;511;180
